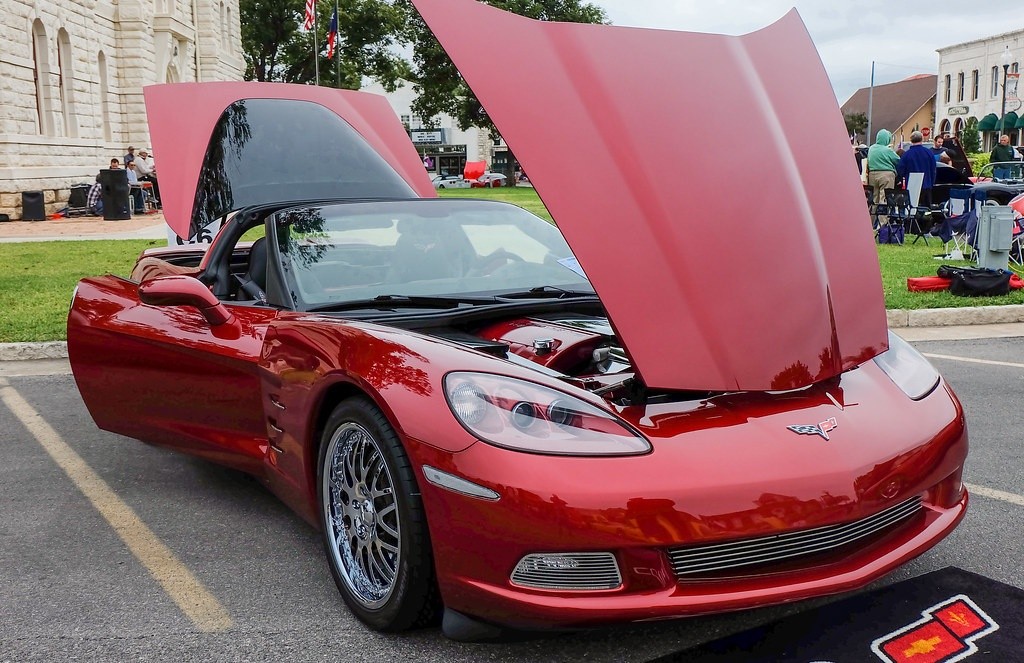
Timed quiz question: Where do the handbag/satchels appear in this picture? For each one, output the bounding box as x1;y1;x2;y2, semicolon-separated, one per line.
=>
874;223;905;243
949;268;1011;295
937;263;980;277
861;157;868;185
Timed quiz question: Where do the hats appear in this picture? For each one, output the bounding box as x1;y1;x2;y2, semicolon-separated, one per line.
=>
139;147;150;154
946;148;957;159
128;145;134;150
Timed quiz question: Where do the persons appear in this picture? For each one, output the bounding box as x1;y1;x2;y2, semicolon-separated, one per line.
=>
87;146;161;216
990;135;1014;179
856;129;951;233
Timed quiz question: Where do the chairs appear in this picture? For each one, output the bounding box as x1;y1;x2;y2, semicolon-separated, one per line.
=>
930;188;1024;267
237;236;324;302
863;184;932;246
384;228;451;284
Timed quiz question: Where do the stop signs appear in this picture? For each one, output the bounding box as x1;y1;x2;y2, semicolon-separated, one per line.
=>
921;128;930;136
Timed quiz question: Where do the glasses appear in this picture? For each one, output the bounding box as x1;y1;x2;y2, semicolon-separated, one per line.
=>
131;164;135;166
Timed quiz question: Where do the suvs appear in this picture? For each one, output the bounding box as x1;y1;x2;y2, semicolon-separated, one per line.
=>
902;141;934;152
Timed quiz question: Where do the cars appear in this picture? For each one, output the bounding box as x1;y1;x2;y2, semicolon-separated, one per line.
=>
471;178;506;188
1013;146;1024;161
64;0;973;633
432;175;472;188
968;161;1024;207
